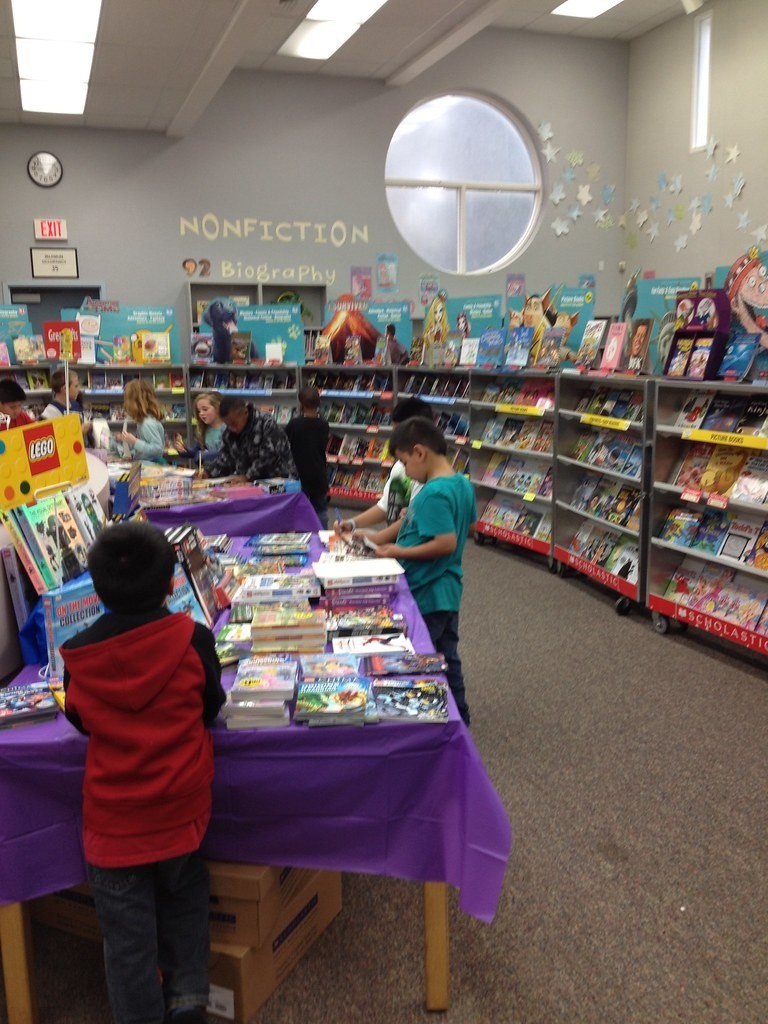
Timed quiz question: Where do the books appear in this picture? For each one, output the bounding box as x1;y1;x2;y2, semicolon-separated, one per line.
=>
0;481;225;627
186;365;467;497
212;530;449;731
656;329;768;636
473;329;564;544
558;319;654;585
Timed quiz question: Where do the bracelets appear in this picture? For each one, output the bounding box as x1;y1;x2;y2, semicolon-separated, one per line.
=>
349;517;357;532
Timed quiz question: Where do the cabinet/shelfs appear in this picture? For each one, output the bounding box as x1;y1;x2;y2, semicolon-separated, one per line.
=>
185;280;330;361
395;367;556;574
298;365;396;503
187;362;300;469
0;363;54;403
558;372;653;618
645;377;768;656
55;363;191;470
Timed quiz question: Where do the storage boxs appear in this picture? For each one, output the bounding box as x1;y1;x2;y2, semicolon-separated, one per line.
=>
29;859;344;1024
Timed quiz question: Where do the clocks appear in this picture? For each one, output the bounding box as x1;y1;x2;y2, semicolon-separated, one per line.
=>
26;150;63;188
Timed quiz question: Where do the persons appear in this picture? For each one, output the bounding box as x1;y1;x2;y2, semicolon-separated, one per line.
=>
62;524;229;1024
0;363;333;531
335;395;480;730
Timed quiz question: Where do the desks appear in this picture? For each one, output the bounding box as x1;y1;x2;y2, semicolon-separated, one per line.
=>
106;454;324;537
0;530;512;1024
